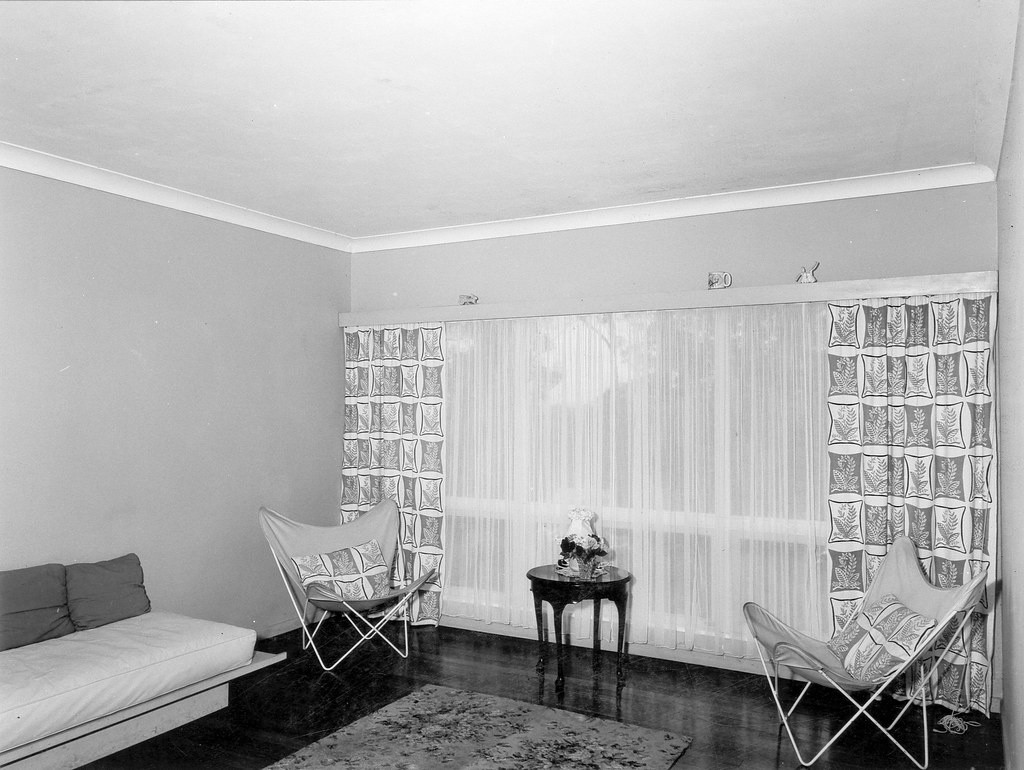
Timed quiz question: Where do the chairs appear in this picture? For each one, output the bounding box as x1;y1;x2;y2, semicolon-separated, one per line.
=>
741;535;989;770
257;497;437;672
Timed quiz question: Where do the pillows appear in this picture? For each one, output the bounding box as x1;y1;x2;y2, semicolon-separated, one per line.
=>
0;563;77;651
65;553;152;634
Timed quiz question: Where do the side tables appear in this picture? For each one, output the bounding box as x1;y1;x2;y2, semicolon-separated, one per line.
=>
526;562;632;690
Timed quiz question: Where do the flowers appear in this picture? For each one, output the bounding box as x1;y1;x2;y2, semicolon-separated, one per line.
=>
559;534;610;565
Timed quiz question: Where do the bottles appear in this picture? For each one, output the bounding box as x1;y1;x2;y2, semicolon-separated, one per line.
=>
565;509;595;572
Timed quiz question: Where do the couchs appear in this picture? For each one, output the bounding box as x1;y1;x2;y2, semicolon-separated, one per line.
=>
0;601;287;770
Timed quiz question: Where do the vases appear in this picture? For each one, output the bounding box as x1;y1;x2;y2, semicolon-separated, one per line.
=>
574;556;597;583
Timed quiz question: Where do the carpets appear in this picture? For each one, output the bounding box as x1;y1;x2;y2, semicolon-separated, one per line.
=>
259;683;693;769
